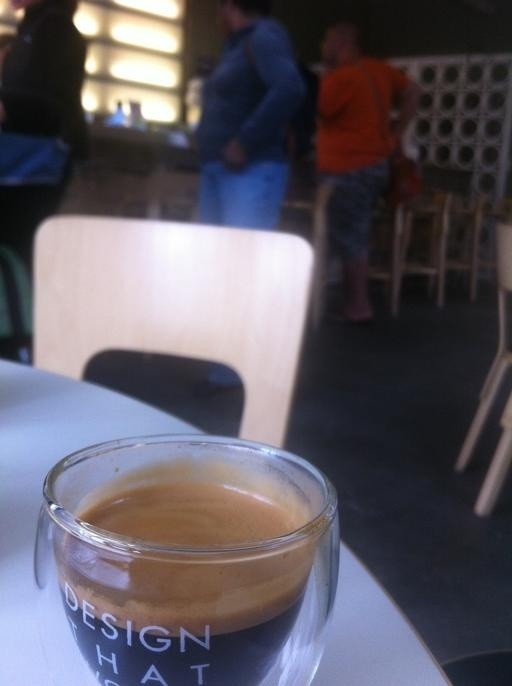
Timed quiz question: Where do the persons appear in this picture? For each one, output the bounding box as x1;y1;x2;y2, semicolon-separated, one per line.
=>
190;2;312;230
2;0;90;360
308;18;421;327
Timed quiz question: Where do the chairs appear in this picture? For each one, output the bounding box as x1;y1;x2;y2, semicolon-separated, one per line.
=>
455;217;512;519
31;212;320;465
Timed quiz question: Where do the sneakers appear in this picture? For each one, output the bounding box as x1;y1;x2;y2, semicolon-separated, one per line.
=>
318;301;374;328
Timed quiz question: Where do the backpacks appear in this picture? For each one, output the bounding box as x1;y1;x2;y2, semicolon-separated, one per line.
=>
283;57;317;161
384;148;422;211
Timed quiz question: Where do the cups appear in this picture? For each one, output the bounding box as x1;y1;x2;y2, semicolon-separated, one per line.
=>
32;432;339;686
